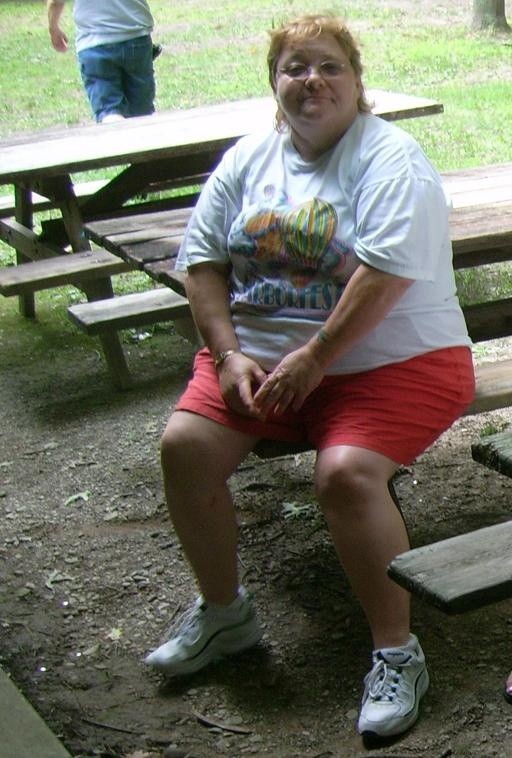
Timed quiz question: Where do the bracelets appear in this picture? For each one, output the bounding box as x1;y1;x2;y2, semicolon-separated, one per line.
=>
213;348;236;368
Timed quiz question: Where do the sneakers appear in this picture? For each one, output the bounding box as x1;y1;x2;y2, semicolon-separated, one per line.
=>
144;589;264;675
357;633;430;738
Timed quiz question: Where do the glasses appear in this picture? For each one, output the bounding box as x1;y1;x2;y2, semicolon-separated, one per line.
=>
279;56;348;80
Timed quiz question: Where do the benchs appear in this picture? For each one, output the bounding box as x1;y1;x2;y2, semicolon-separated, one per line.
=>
459;360;511;415
67;286;192;337
383;521;509;605
2;248;131;299
1;180;109;218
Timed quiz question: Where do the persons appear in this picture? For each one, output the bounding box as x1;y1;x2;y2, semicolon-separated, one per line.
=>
44;1;166;124
139;13;485;745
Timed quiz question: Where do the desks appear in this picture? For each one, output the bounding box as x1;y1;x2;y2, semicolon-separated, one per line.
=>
0;87;447;377
82;160;511;297
470;429;510;479
